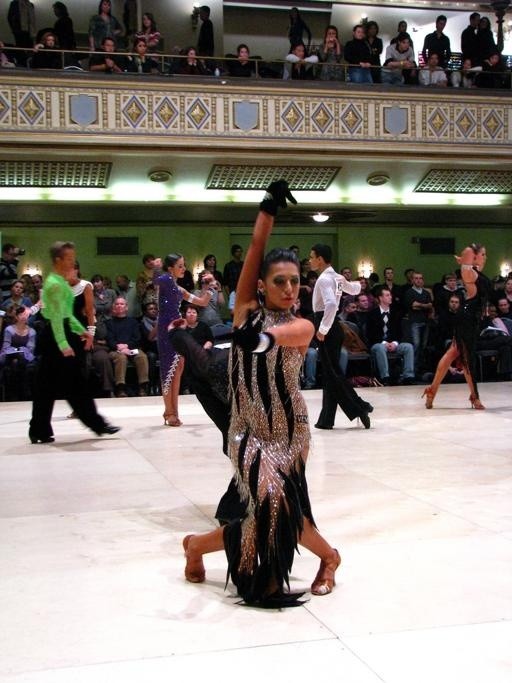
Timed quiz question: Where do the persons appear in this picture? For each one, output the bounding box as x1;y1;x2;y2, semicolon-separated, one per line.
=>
26;240;121;443
163;318;230;449
304;243;376;430
0;0;214;75
225;44;261;77
151;252;215;425
18;258;96;419
1;242;511;396
182;176;346;609
421;241;498;410
284;7;505;90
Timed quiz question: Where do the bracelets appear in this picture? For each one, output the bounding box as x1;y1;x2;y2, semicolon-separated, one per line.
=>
86;325;97;336
29;304;40;315
207;281;218;295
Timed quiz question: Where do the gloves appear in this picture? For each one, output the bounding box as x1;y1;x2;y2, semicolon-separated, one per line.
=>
232;319;274;355
259;179;297;216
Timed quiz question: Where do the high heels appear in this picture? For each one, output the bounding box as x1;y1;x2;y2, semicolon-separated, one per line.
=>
183;535;204;582
311;549;340;594
422;388;434;409
470;395;484;409
163;413;181;426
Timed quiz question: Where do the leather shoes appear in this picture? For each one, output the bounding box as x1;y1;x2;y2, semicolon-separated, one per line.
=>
315;424;332;429
31;436;54;444
360;402;373;429
98;427;119;435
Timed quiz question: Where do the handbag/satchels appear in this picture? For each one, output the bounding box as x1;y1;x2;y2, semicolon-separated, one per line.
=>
349;376;383;387
